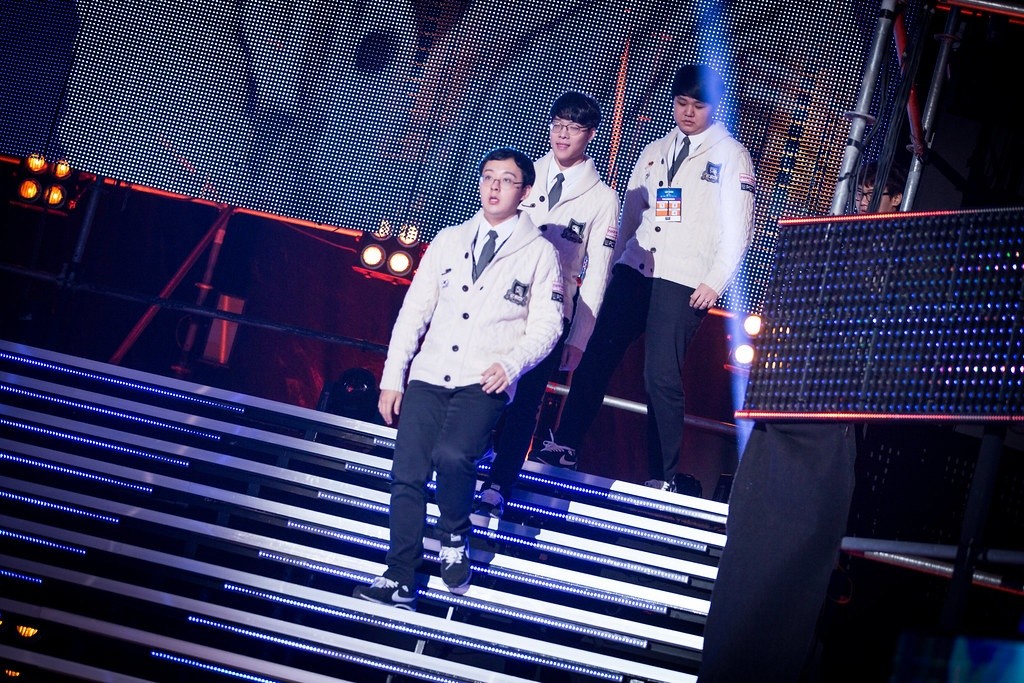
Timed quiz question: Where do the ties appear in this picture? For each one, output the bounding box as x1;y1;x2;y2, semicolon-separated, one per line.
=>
476;230;498;277
669;137;691;181
547;173;565;211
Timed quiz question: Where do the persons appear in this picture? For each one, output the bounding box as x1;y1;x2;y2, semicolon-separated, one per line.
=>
351;148;564;615
59;1;903;299
527;66;756;490
853;155;905;214
481;90;622;520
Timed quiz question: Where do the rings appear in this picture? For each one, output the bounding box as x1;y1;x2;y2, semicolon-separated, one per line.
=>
705;300;708;303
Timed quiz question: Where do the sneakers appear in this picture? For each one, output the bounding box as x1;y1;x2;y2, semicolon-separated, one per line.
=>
352;576;417;611
474;481;507;518
438;531;471;588
528;428;577;471
645;475;677;492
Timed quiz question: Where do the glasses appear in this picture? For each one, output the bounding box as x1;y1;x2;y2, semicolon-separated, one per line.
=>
480;175;524;190
854;191;893;203
549;121;589;136
